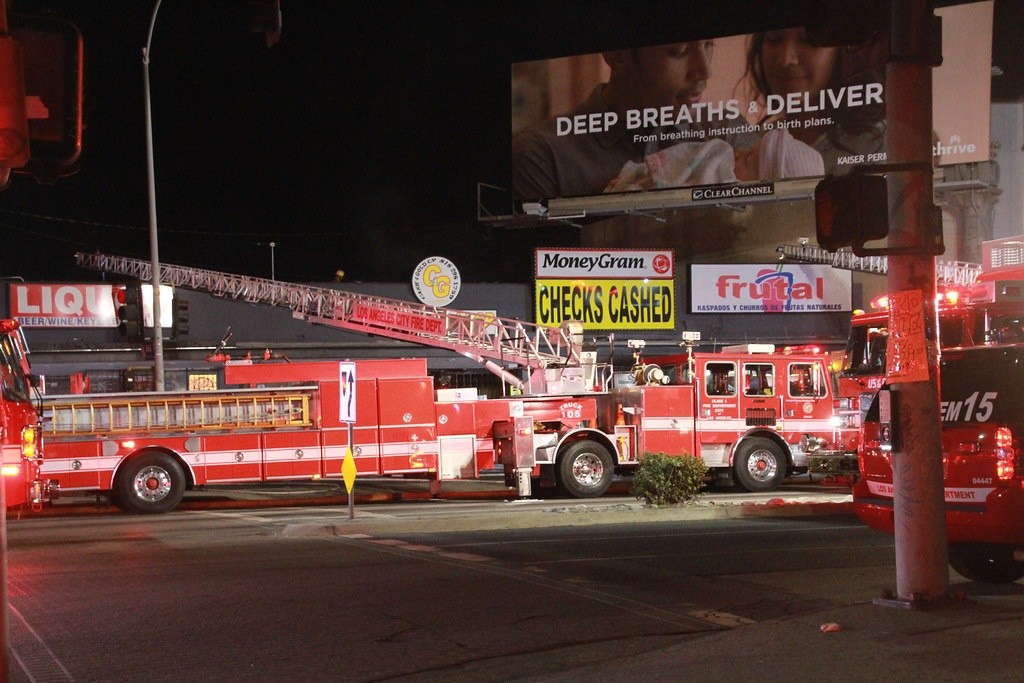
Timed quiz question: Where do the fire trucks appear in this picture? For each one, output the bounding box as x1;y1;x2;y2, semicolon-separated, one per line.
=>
63;242;842;497
0;311;499;520
839;236;1024;588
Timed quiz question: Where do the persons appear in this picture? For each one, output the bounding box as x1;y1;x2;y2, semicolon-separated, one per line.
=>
515;37;759;206
604;129;828;195
731;22;942;173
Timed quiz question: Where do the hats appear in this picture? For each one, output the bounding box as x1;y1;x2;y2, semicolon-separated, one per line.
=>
758;126;826;181
